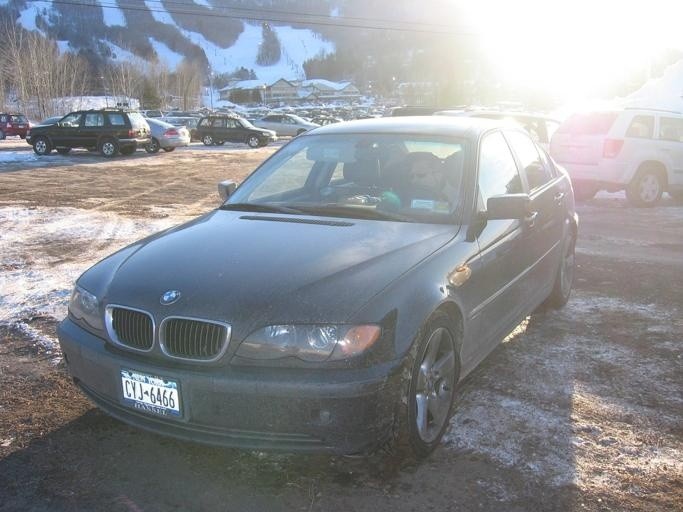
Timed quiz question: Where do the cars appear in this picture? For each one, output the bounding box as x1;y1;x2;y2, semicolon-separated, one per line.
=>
196;114;277;148
39;116;76;125
49;109;584;475
139;101;392;139
431;108;564;151
87;118;191;154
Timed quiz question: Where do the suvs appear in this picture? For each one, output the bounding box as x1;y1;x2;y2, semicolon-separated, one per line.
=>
25;108;152;159
548;106;682;208
0;112;30;140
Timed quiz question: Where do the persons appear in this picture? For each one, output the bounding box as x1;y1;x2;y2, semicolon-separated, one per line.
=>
400;150;453;213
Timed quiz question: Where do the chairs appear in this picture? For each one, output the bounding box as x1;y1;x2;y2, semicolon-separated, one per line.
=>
330;147;462;201
